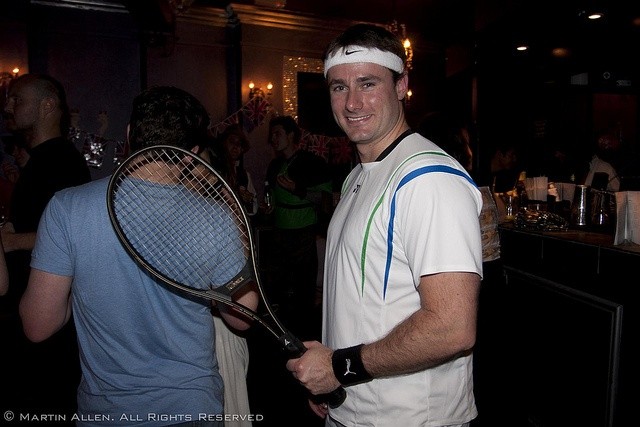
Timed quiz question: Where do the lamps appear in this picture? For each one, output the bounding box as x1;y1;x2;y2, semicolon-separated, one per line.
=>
249;81;273;105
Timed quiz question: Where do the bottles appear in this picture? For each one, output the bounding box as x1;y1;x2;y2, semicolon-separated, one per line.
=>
506;196;513;215
577;185;589;226
263;180;273;211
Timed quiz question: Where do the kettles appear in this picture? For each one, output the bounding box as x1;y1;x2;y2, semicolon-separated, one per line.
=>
590;187;616;232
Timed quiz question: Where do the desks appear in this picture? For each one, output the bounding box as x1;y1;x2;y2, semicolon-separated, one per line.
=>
494;222;640;427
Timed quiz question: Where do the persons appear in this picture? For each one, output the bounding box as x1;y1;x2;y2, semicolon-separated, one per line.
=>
1;73;92;425
256;116;336;337
1;135;31;219
285;23;485;427
16;85;261;427
179;136;228;197
216;122;259;231
1;235;11;298
552;138;622;193
475;140;518;213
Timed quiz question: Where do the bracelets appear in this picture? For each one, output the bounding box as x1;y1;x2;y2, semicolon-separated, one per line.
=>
331;343;373;388
